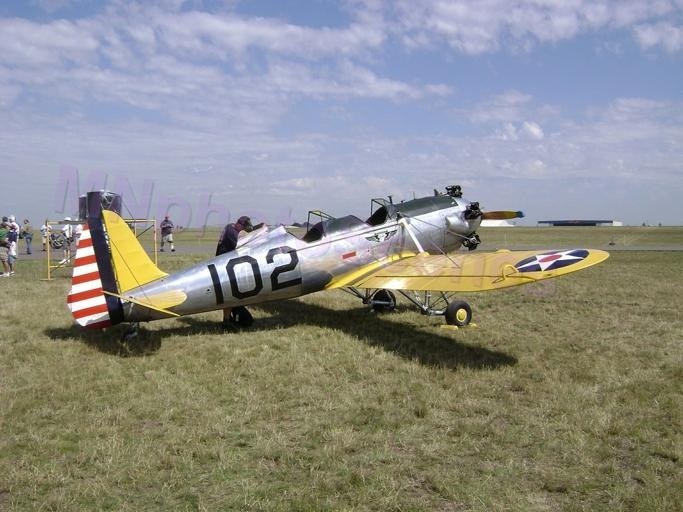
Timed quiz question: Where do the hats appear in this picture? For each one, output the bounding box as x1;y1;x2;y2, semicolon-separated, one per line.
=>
238;216;253;227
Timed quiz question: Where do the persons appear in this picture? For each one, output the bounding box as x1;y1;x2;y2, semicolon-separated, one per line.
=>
160;215;176;252
0;215;83;277
216;216;253;332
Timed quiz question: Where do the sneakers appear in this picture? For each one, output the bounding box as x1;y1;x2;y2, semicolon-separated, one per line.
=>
0;271;16;277
59;259;69;264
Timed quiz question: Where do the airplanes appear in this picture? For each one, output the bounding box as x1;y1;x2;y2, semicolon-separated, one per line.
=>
65;184;612;349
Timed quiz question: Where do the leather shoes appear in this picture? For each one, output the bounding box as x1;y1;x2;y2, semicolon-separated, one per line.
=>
220;321;236;331
